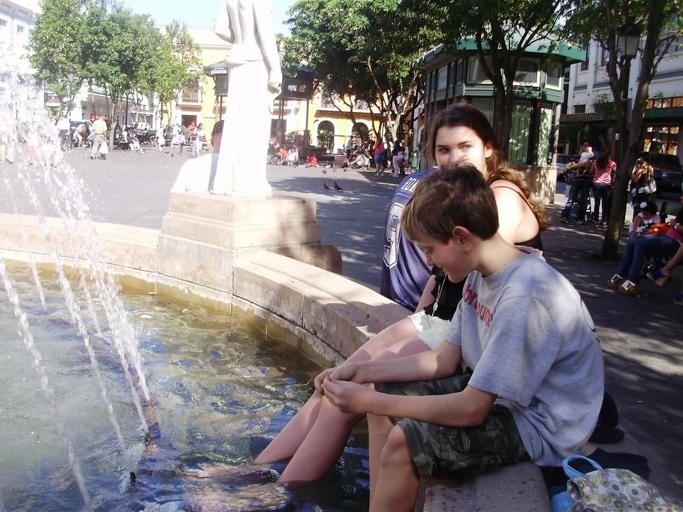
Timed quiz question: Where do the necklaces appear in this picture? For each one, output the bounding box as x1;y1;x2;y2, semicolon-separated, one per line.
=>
431;272;447;316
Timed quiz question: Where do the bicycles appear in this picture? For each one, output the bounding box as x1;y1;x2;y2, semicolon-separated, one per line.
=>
59;134;74;152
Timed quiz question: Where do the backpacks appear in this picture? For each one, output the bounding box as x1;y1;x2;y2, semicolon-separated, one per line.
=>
546;449;683;512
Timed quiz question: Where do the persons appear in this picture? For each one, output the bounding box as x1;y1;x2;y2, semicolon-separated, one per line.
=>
255;105;549;480
560;142;616;227
379;164;439;313
315;165;604;511
628;153;657;219
342;137;407;178
0;114;224;193
607;201;681;294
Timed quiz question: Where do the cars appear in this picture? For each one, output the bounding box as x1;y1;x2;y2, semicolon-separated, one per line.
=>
548;152;572;176
69;120;206;150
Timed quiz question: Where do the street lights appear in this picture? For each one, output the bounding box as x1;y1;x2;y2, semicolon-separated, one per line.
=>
614;14;645;130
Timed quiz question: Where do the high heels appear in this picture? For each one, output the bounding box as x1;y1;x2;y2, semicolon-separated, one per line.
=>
671;292;682;306
645;268;669;287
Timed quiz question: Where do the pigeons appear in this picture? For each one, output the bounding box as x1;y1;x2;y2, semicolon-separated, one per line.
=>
324;182;335;191
334;181;344;191
321;169;329;174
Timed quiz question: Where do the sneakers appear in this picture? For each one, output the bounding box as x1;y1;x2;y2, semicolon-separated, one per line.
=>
605;274;625;288
559;215;609;228
614;280;639;296
